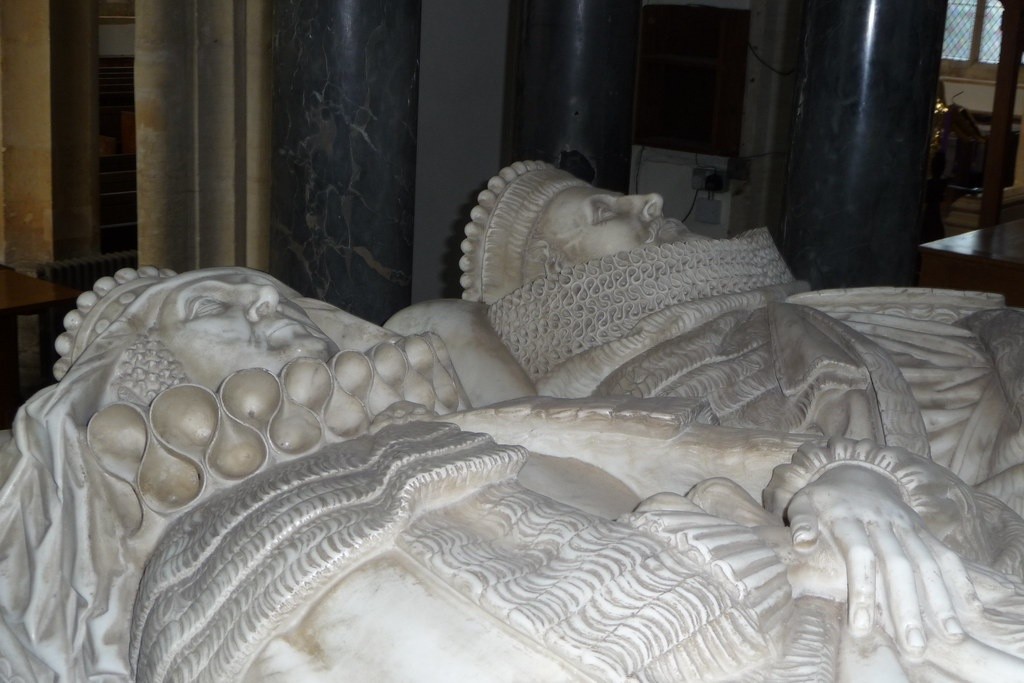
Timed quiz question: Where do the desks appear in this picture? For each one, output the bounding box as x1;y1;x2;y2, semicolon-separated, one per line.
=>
917;215;1024;310
0;264;87;430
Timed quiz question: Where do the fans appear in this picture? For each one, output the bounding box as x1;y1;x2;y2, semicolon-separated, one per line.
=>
36;248;138;378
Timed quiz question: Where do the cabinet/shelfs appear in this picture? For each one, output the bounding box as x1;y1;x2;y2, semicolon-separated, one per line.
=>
631;2;752;158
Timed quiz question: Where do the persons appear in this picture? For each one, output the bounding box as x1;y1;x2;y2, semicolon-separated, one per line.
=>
0;160;1024;683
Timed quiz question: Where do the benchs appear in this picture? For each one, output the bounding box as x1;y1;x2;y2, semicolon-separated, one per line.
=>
934;75;1024;234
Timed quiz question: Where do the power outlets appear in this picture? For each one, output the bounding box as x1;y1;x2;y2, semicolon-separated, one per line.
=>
690;166;723;226
